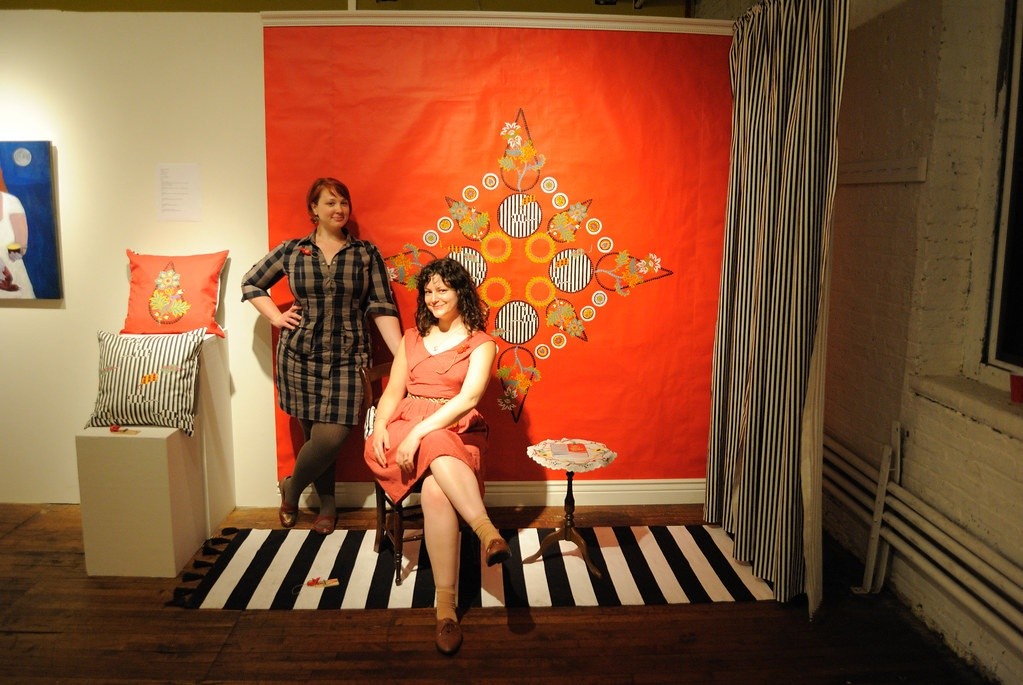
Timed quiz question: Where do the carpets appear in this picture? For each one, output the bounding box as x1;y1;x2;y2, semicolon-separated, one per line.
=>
162;524;778;612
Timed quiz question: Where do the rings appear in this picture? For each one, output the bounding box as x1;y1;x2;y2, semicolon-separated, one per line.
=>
403;458;408;463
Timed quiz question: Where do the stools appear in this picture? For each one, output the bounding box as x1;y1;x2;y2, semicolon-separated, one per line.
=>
522;438;618;577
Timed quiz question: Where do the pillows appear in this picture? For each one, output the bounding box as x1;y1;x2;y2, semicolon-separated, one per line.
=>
119;248;229;339
84;326;208;438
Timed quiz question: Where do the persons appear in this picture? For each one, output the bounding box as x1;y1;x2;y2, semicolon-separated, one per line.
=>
364;259;513;654
241;178;402;535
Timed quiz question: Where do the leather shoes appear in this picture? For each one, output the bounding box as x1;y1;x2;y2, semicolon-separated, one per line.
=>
435;606;463;653
313;512;337;534
278;475;298;528
486;537;512;567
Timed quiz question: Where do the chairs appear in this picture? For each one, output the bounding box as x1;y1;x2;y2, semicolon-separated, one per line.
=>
358;361;478;586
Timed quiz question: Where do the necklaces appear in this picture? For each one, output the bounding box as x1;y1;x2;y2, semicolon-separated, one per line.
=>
430;325;464;351
